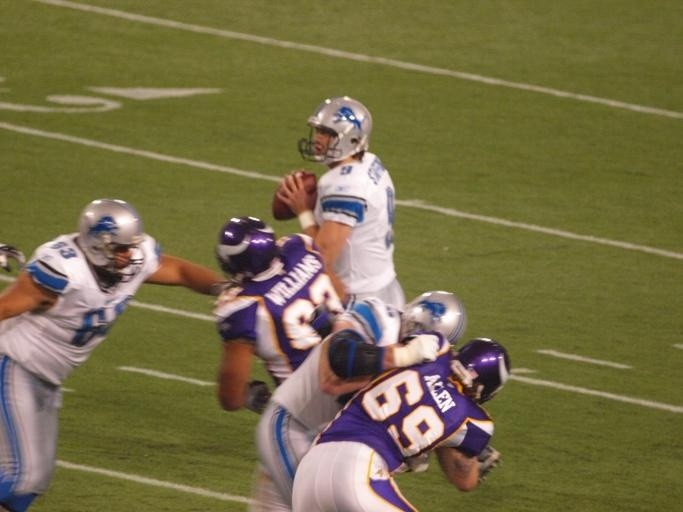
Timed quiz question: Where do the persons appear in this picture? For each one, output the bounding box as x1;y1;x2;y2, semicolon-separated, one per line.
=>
275;95;502;482
252;290;467;512
291;331;511;512
214;216;341;512
0;198;236;511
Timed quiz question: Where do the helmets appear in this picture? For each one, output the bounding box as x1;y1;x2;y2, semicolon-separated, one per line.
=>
214;216;275;278
450;338;511;405
401;289;466;346
78;197;143;267
308;96;373;166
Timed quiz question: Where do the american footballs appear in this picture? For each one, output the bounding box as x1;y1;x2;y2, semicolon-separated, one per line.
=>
270;172;317;220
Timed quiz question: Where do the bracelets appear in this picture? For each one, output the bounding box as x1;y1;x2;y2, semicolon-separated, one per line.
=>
296;209;316;230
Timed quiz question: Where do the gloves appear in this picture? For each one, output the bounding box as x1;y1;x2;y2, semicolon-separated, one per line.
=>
393;333;440;369
244;380;271;414
